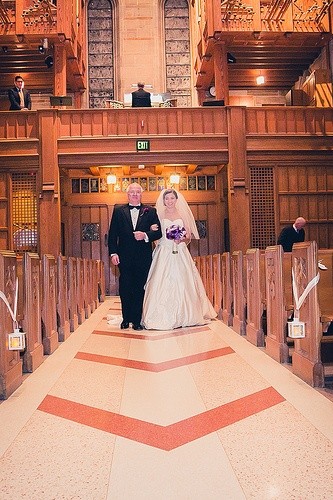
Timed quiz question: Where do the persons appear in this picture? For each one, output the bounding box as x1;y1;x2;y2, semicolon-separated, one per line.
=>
131;82;152;107
8;76;31;110
103;189;217;329
277;217;306;252
108;183;161;330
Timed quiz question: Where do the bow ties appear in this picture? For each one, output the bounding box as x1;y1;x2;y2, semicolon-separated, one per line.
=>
129;205;141;210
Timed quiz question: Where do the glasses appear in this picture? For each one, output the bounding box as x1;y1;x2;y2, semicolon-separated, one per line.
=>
16;81;23;83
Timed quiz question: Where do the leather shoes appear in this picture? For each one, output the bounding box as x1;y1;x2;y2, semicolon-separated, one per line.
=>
132;323;143;330
121;321;129;328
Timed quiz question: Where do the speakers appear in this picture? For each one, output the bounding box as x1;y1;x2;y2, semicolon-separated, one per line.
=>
227;52;236;63
45;55;53;68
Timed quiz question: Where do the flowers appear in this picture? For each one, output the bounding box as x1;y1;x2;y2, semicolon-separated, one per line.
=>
141;208;148;217
166;225;186;254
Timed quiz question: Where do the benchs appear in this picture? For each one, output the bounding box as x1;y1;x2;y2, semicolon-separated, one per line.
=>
0;250;105;401
193;241;333;388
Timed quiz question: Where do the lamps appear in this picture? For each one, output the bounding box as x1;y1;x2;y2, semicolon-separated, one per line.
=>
39;46;44;52
4;48;7;52
170;166;180;184
9;323;26;350
45;54;54;67
131;165;155;177
257;70;264;85
228;52;236;63
107;167;116;185
287;318;305;338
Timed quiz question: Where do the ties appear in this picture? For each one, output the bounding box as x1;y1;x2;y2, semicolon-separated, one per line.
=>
296;229;299;233
19;89;21;93
